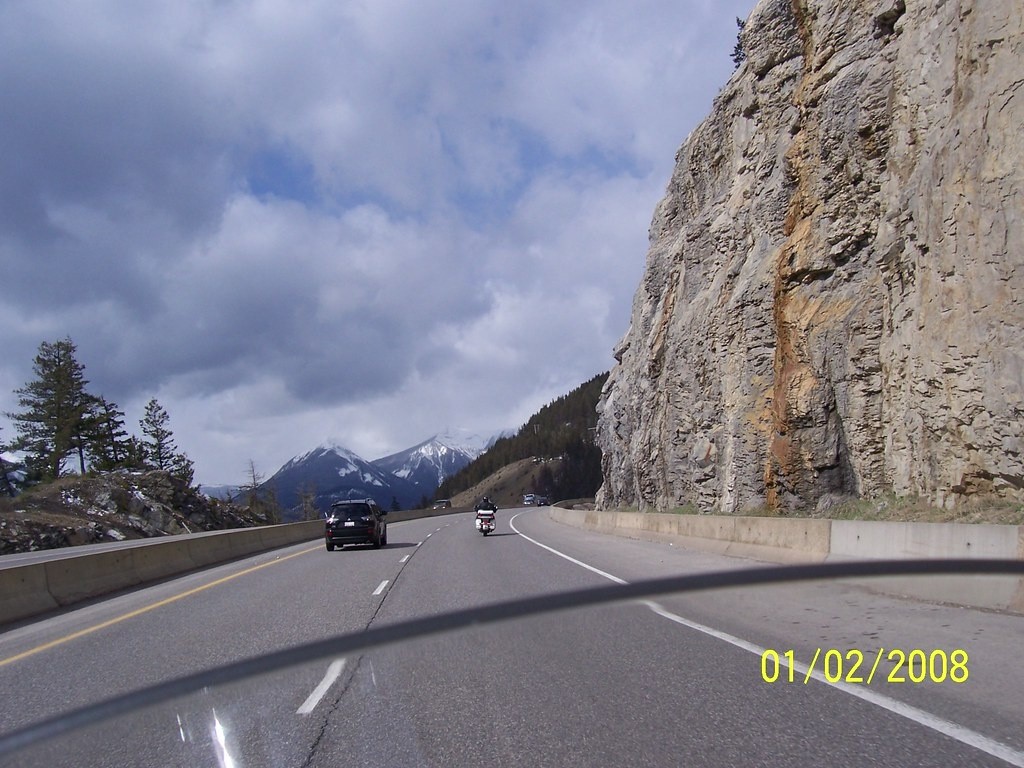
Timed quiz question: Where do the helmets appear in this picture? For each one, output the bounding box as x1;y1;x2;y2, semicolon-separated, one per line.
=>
483;497;488;501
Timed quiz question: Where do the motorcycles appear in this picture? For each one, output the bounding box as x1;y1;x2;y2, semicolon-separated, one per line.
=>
474;503;498;537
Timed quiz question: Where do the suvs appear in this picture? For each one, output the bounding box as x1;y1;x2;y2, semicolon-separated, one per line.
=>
325;497;388;551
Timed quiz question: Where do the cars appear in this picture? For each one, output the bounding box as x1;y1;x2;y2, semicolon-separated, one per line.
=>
537;496;551;506
523;494;535;505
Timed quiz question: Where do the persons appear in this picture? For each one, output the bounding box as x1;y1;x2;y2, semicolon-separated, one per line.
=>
475;497;498;513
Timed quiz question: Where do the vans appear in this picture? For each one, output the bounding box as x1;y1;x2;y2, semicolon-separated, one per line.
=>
432;500;451;511
